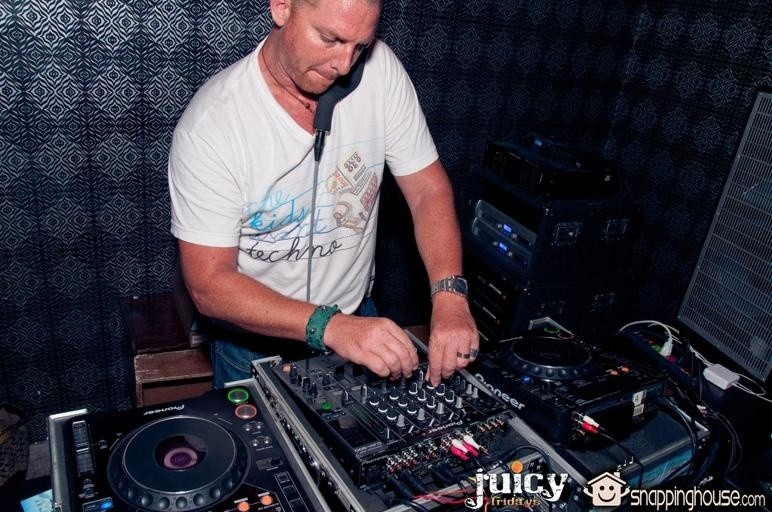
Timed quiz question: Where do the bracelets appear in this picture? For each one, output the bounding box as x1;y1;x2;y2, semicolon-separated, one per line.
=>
303;302;344;358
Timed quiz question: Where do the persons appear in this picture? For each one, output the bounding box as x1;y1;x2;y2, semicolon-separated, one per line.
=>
164;0;483;390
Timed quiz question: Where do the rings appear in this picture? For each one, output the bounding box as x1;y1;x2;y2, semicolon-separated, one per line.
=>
456;351;472;361
469;347;480;358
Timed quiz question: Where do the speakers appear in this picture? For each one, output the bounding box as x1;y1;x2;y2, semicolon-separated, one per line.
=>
676;88;772;383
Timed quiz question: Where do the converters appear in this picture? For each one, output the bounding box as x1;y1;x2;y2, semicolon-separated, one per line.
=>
703;363;740;391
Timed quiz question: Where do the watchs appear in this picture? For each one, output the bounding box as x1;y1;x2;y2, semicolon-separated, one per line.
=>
427;274;473;300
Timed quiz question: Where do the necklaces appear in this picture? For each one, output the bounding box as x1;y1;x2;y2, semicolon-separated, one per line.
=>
260;45;316;120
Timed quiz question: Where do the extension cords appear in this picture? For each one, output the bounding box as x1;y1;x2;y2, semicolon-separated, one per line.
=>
627;328;692;383
